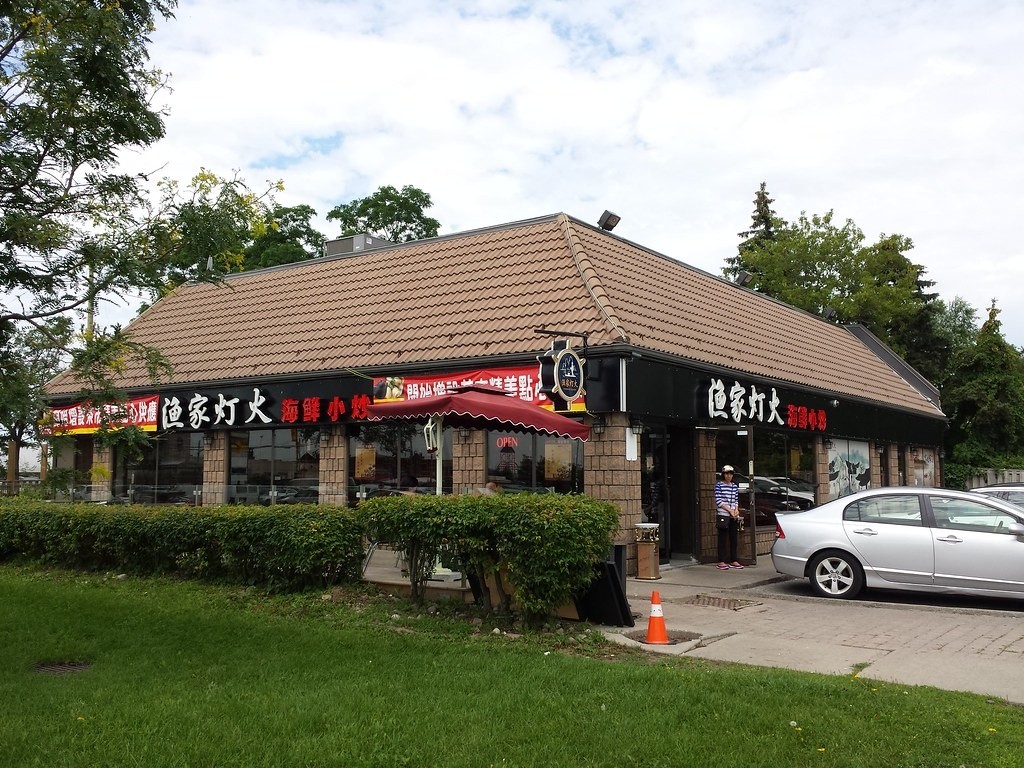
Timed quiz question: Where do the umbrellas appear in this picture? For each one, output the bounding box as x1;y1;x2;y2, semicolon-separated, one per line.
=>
367;382;591;495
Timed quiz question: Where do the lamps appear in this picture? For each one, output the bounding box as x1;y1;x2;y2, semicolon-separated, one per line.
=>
319;427;332;441
876;442;884;454
824;436;833;449
203;430;213;445
592;416;605;434
459;426;470;437
597;210;621;232
631;416;644;435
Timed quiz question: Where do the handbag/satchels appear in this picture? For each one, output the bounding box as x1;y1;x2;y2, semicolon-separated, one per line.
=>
715;515;730;529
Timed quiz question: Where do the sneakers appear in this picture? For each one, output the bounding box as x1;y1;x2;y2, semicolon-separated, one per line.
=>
716;562;744;570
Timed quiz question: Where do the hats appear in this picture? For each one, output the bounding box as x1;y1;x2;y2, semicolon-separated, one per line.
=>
723;465;734;472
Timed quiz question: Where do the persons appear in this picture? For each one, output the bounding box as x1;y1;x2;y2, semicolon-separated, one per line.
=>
715;465;744;570
400;475;419;493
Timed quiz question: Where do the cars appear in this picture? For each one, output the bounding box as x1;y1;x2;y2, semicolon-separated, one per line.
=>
871;482;1024;533
715;471;815;525
769;486;1024;601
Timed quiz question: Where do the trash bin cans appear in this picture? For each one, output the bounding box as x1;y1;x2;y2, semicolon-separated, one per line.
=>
634;522;662;579
725;516;746;560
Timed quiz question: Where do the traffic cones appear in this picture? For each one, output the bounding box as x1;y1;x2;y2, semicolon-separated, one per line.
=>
641;589;677;646
